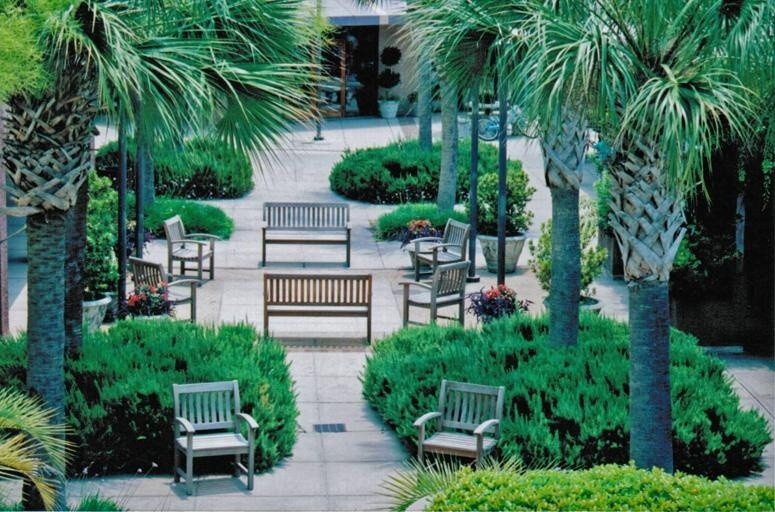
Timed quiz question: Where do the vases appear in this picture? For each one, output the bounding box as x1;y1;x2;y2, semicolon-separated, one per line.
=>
126;314;167;322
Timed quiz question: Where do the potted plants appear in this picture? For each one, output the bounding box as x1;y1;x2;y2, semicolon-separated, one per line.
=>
464;169;534;272
528;199;605;315
81;169;119;332
376;47;403;119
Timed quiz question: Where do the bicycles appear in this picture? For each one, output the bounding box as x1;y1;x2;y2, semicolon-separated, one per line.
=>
478;104;541;141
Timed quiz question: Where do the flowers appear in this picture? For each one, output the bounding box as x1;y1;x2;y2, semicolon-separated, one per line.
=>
130;283;169;313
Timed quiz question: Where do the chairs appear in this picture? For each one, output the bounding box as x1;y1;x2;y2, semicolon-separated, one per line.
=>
412;379;506;469
125;255;198;324
408;218;471;280
166;379;258;495
398;261;467;327
156;215;219;286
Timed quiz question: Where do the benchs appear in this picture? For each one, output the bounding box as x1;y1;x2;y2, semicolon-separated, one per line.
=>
262;272;374;344
261;202;353;267
